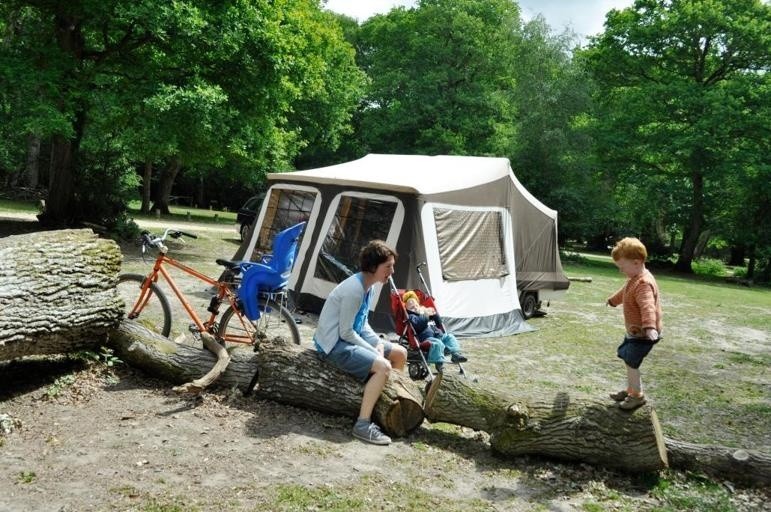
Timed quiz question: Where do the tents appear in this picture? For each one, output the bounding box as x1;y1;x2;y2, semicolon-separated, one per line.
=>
220;150;570;342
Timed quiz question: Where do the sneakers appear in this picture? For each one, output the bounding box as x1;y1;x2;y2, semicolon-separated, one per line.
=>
436;364;442;371
451;356;467;363
352;421;391;445
610;391;647;411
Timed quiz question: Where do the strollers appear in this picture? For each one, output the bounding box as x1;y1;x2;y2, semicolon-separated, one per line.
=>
389;260;481;395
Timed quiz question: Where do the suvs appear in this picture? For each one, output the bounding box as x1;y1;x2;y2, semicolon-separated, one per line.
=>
235;196;311;251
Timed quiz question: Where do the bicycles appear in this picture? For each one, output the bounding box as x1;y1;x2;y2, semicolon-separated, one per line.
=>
110;227;301;357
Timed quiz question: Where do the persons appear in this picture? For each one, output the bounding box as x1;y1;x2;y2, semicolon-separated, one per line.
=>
312;239;408;446
402;289;467;371
605;234;663;413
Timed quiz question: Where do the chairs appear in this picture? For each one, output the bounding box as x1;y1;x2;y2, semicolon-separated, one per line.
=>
235;221;307;320
